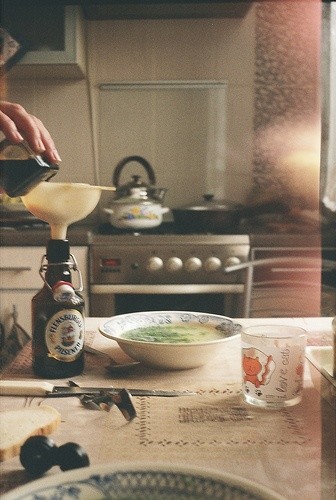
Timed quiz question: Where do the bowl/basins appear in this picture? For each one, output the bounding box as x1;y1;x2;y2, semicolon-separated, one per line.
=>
98;311;245;371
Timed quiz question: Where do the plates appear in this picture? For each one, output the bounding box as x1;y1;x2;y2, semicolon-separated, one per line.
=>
0;460;289;500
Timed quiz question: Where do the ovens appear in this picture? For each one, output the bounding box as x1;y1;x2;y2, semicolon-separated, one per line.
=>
85;220;251;318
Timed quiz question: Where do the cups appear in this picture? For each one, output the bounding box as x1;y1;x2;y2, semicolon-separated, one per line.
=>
240;325;309;409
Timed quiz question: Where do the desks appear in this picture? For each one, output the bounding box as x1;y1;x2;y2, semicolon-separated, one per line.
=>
0;315;336;500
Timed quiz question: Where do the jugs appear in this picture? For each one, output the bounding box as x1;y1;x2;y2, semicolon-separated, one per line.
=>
112;155;168;204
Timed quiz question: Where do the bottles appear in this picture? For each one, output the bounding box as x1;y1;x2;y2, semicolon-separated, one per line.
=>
31;239;86;379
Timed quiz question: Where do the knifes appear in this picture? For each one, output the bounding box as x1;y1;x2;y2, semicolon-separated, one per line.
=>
0;380;199;397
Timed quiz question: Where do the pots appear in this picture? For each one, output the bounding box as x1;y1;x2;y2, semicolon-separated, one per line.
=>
171;194;290;235
100;186;170;229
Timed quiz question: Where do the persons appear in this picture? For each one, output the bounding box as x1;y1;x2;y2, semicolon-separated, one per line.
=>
0;101;62;163
